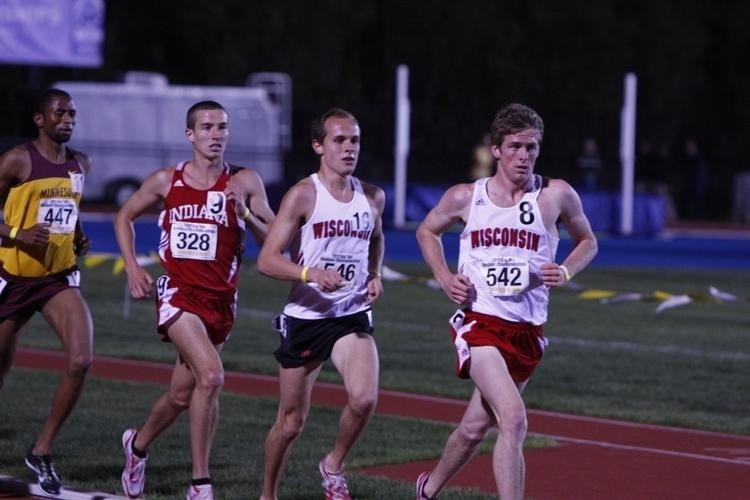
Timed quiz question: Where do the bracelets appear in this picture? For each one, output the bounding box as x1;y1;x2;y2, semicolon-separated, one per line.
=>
300;265;310;284
240;207;249;220
560;265;572;283
9;226;19;241
369;268;382;277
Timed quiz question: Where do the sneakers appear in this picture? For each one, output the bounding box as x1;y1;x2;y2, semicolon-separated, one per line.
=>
186;484;217;498
24;445;64;495
121;429;148;497
319;457;353;499
415;471;432;499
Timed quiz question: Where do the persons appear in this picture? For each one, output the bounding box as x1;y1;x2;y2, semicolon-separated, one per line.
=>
0;88;97;495
469;131;495;184
577;136;602;188
255;109;387;500
415;103;600;500
113;100;278;500
